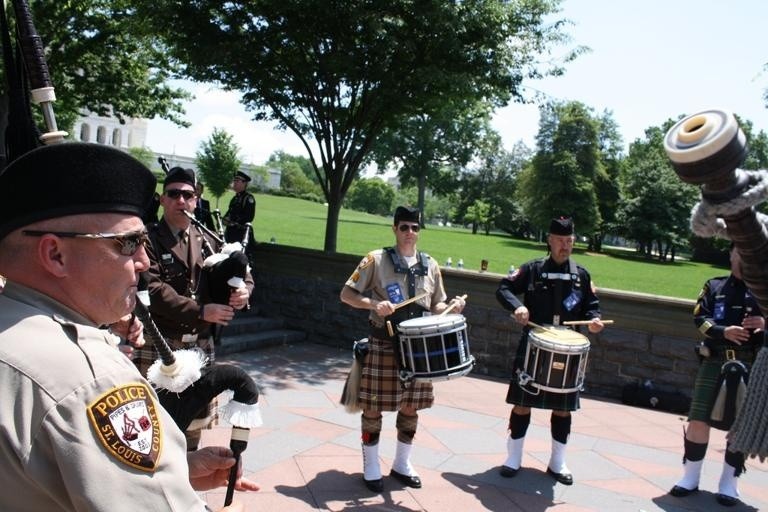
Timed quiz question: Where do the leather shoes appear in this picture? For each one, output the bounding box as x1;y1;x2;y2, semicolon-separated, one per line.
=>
668;479;700;497
362;476;383;494
501;457;522;478
389;468;422;488
547;465;574;485
717;492;739;505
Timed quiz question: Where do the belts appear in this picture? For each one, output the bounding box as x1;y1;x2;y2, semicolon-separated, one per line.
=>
704;348;761;360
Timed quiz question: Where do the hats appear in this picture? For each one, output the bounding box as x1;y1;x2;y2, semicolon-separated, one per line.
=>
163;165;197;186
550;220;575;236
234;170;252;183
1;141;158;241
394;205;420;224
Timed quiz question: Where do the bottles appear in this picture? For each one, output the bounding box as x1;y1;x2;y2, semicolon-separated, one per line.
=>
509;264;515;275
457;258;464;270
445;257;452;268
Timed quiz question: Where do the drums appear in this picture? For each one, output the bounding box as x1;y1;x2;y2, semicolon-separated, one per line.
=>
518;323;593;400
396;312;477;385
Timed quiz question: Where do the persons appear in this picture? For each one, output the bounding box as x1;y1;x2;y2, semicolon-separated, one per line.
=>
111;313;145;359
339;205;466;493
133;167;255;453
672;240;768;506
496;218;604;486
0;142;259;512
221;171;255;311
194;182;217;232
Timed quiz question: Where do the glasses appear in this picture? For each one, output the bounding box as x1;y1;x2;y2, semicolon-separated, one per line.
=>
397;224;420;233
161;188;198;200
23;226;151;256
